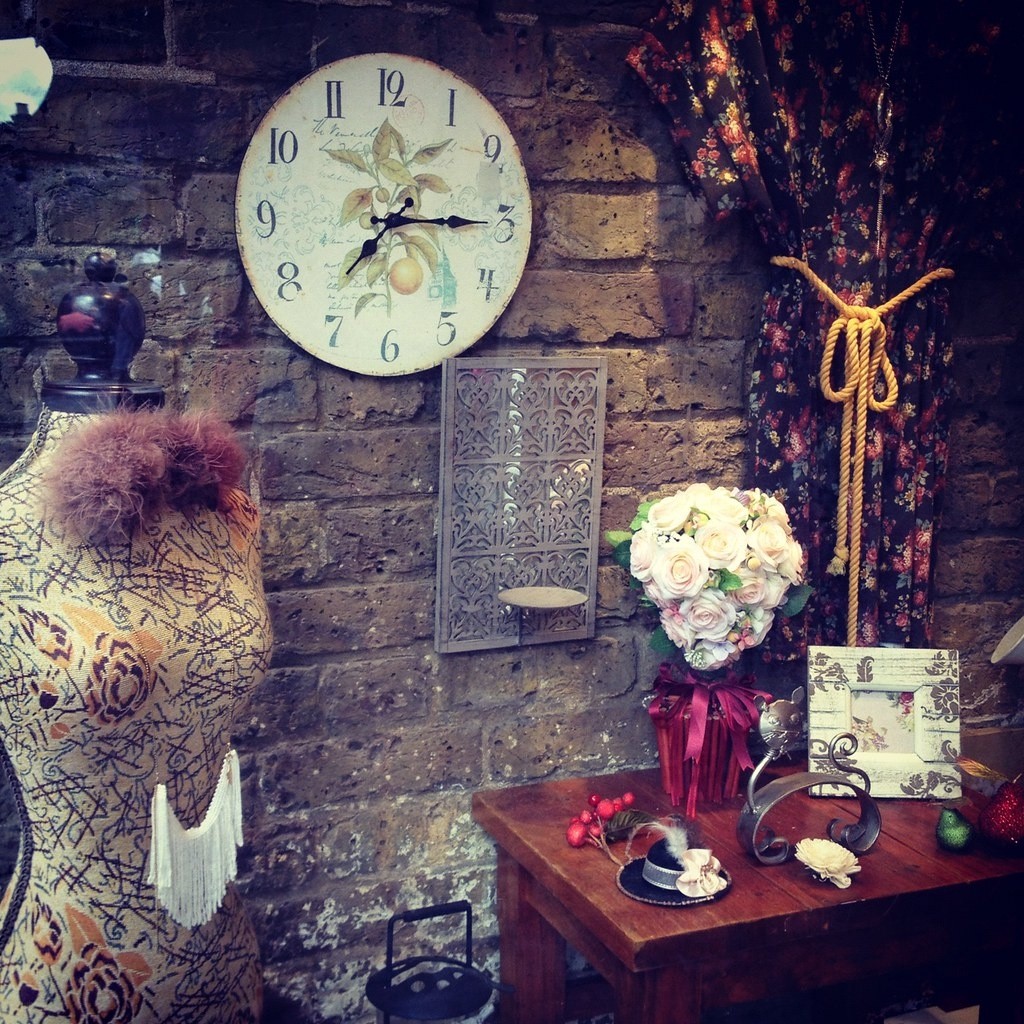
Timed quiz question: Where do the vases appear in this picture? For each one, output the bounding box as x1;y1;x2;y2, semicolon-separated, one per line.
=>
640;661;773;818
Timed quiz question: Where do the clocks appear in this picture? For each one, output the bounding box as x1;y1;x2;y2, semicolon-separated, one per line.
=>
233;52;532;378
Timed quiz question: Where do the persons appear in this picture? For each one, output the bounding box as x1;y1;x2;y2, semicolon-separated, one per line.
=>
0;409;276;1024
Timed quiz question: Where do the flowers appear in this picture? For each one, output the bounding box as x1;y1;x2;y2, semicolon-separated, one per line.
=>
605;479;814;675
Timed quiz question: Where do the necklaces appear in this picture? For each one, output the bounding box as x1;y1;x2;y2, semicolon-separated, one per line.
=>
31;431;244;930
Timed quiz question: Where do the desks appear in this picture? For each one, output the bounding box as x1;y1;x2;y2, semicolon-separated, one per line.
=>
471;727;1024;1024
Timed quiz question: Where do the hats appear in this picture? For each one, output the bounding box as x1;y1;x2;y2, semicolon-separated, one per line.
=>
616;822;733;907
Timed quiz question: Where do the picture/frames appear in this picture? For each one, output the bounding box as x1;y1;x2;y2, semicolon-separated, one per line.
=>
807;645;961;799
